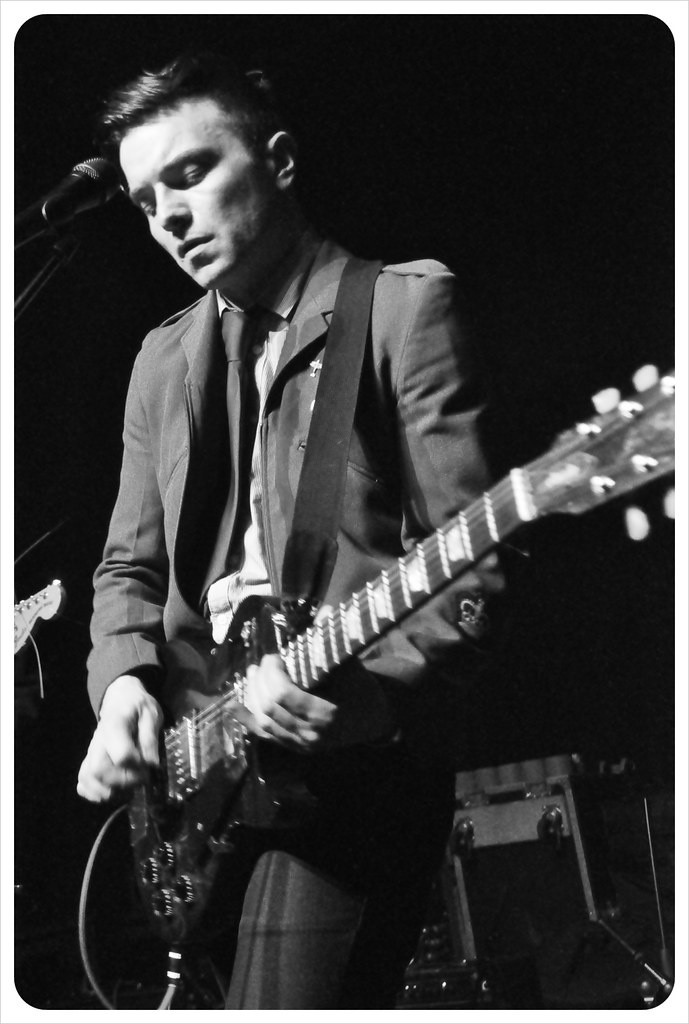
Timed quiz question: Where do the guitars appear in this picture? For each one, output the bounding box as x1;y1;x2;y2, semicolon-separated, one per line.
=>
126;363;675;941
14;578;67;657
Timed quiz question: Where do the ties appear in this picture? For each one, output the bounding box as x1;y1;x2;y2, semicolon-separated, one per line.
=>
196;307;282;612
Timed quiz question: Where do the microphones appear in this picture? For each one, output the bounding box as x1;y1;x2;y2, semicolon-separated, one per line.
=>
14;156;122;247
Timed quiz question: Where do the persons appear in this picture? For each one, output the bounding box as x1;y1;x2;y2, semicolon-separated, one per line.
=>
77;46;526;1010
